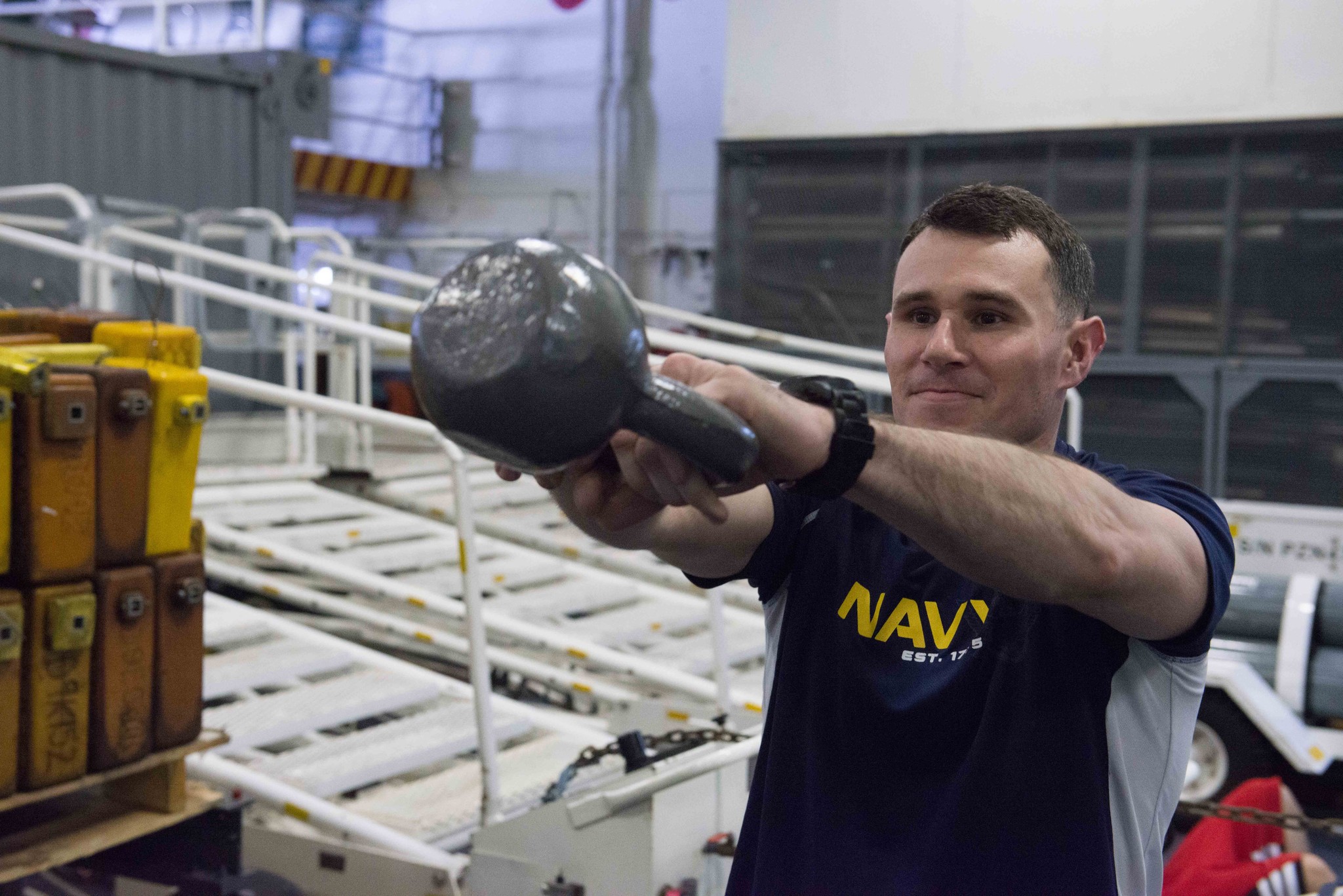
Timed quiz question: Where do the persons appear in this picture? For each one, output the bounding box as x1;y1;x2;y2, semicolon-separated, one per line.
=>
1160;776;1343;896
491;180;1236;896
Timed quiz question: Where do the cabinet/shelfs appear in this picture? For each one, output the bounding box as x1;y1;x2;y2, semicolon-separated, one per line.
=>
708;115;1343;507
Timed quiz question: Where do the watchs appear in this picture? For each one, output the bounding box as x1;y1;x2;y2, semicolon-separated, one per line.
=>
773;376;877;517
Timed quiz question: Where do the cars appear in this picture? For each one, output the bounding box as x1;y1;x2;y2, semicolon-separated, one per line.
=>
1172;494;1343;844
